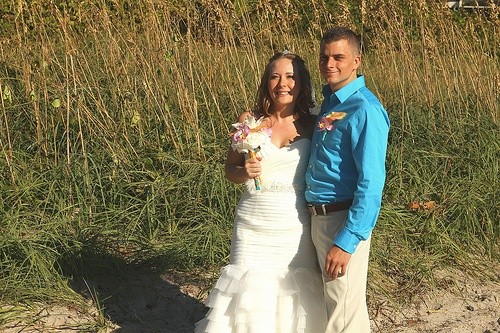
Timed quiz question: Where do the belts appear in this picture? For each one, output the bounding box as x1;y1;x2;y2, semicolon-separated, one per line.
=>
307;198;353;215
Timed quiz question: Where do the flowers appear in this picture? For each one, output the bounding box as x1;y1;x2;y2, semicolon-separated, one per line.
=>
230;114;272;190
317;111;347;140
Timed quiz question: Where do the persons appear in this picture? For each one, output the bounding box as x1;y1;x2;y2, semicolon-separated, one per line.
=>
304;26;392;333
193;50;328;333
